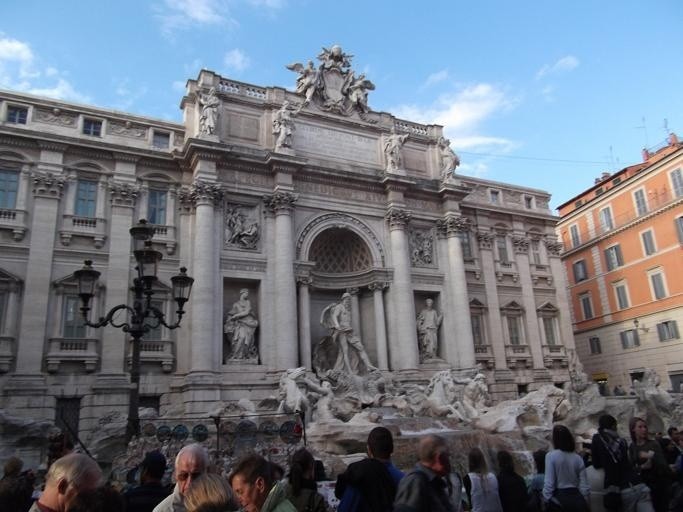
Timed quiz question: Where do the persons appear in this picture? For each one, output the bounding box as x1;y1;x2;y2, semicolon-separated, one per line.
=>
328;292;378;376
679;380;683;393
195;87;220;135
348;72;376;113
294;59;320;103
598;381;637;396
451;372;487;418
224;286;260;360
437;135;459;182
382;132;410;170
272;99;304;148
294;373;334;422
417;298;443;359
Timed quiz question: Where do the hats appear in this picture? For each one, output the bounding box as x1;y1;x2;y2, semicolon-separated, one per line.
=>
291;448;314;463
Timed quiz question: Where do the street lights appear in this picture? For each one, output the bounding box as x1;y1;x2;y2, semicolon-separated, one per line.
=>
71;216;196;446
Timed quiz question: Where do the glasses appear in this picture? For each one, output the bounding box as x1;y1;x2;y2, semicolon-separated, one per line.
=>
177;470;201;481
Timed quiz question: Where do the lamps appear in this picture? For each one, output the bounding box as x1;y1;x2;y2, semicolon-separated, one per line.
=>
633;317;650;333
